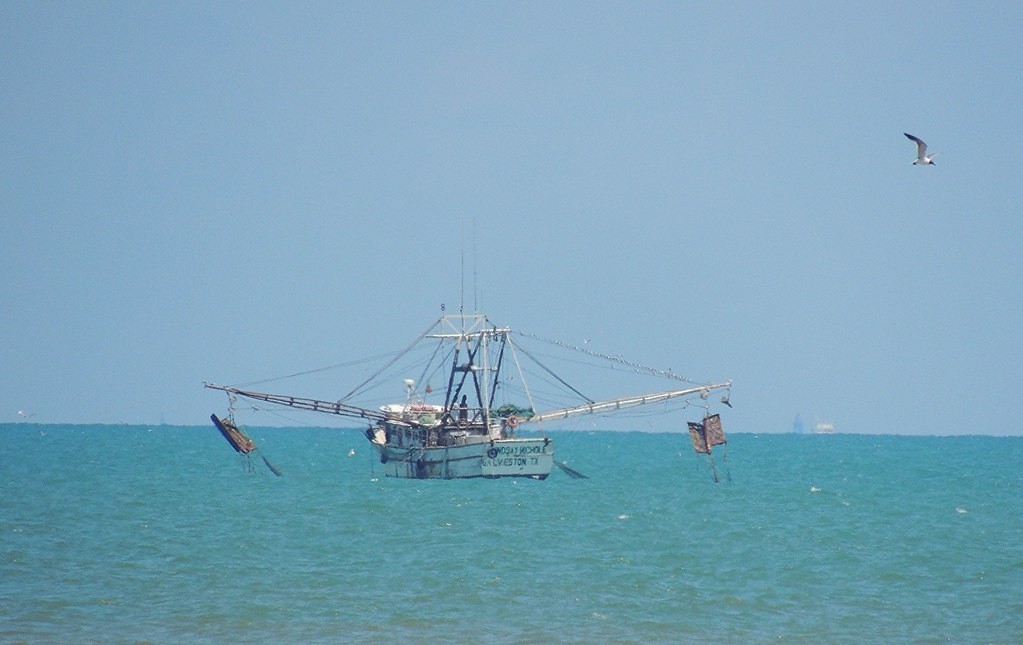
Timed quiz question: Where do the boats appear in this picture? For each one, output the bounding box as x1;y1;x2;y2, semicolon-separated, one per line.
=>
201;305;738;479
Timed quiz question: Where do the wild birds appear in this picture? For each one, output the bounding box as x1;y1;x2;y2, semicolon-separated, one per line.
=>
583;338;591;344
903;131;945;166
16;408;24;417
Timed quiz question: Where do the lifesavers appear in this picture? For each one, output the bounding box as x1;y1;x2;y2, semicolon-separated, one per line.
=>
486;447;498;459
508;415;519;428
380;454;388;464
417;457;424;470
